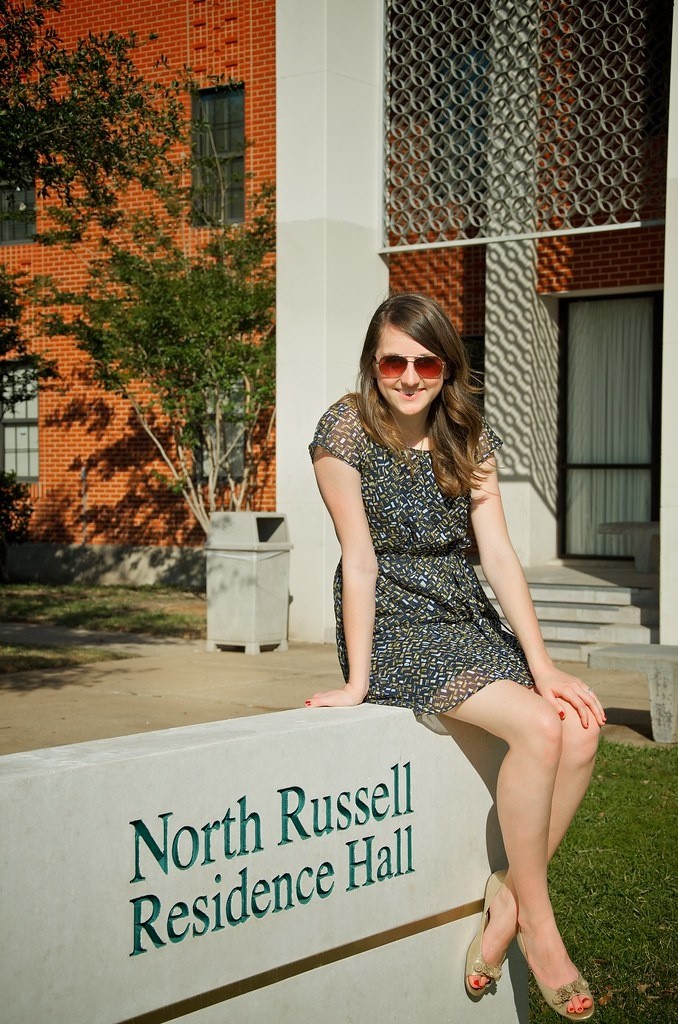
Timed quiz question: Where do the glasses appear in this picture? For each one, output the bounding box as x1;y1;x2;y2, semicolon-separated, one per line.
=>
373;354;446;379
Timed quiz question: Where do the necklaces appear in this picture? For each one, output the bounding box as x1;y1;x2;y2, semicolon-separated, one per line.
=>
389;408;424;454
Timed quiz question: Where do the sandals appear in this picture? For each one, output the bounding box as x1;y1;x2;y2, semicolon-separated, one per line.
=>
465;868;518;997
516;925;595;1021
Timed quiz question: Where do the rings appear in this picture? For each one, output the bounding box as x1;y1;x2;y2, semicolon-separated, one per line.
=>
587;687;592;694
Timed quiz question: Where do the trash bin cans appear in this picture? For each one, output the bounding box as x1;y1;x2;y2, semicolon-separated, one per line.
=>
207;512;295;647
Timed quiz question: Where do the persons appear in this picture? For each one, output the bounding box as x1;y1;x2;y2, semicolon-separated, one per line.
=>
303;294;606;1022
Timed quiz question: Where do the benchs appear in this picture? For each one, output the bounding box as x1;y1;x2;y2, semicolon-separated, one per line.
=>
579;640;678;750
